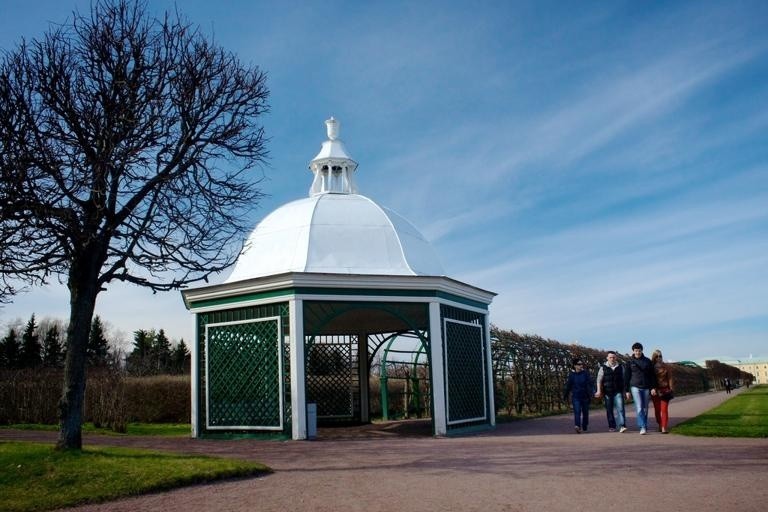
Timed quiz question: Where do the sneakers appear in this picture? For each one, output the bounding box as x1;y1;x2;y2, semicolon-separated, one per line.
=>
662;430;668;434
640;429;646;434
574;426;581;433
620;426;627;433
609;427;614;431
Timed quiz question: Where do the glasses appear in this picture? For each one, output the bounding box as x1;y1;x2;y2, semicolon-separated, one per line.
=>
576;363;584;366
655;355;662;358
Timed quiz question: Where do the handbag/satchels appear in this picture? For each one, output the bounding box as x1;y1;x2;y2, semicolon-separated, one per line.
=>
661;392;673;401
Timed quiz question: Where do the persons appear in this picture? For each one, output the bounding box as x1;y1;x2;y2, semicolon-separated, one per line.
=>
594;350;631;433
622;342;657;435
650;348;676;432
562;357;600;434
724;374;732;394
